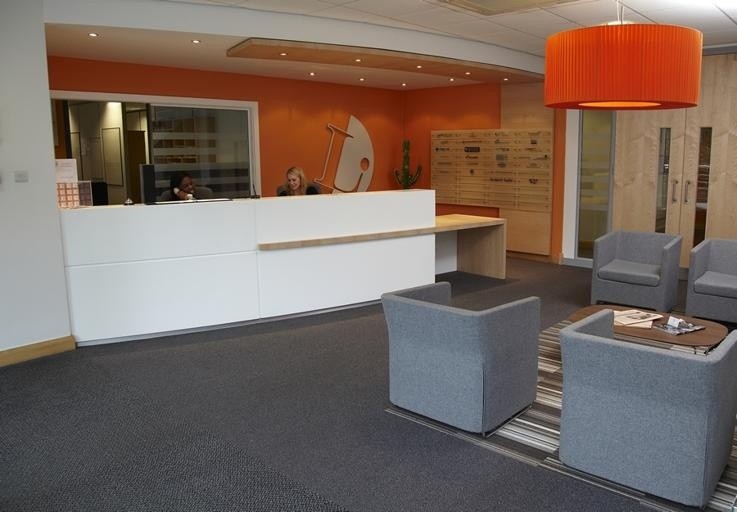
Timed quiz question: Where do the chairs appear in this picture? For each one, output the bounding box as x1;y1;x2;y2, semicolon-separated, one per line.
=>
585;226;682;316
157;185;215;200
554;307;735;512
275;179;323;194
685;237;735;325
379;278;544;439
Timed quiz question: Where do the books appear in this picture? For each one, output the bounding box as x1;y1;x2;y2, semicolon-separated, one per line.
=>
614;309;706;336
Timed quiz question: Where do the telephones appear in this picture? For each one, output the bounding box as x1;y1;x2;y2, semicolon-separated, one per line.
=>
173;187;193;200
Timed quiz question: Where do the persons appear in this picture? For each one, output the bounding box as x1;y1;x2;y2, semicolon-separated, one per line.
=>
279;167;319;195
170;174;196;200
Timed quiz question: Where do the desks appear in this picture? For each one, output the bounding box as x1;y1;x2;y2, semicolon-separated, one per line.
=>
436;211;510;281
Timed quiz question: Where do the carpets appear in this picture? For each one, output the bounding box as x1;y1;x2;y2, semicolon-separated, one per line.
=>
380;321;736;512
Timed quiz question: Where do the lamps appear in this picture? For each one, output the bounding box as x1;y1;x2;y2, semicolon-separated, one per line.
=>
540;1;704;113
